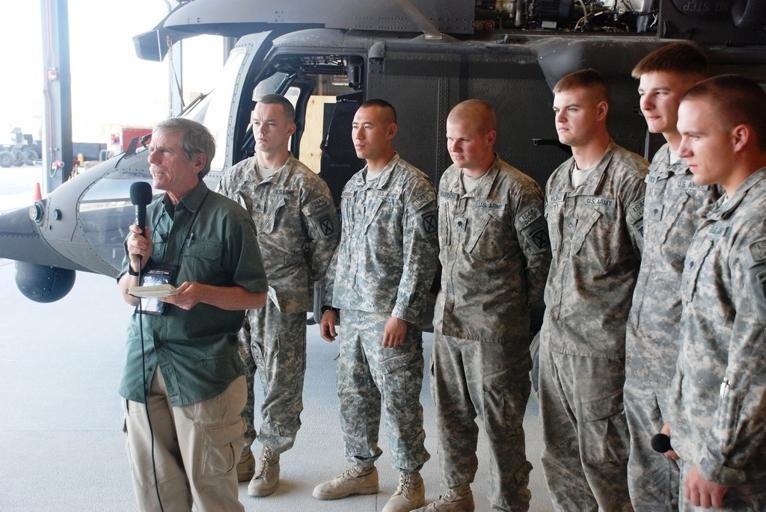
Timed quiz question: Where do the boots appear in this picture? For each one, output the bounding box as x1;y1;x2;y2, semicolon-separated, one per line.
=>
424;483;474;511
247;448;279;497
236;451;255;482
381;473;425;512
312;464;379;501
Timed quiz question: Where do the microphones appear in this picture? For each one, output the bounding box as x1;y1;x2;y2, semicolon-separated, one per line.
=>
130;181;152;259
650;434;671;453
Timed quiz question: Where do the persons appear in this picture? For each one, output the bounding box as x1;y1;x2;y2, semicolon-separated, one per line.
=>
530;67;649;511
409;99;554;511
313;99;439;511
115;116;269;511
211;95;338;496
621;42;724;510
674;72;766;510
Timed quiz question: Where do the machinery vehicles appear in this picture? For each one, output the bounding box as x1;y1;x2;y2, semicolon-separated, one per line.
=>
0;129;107;168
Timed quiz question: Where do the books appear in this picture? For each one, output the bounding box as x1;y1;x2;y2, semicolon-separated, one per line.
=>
127;284;177;298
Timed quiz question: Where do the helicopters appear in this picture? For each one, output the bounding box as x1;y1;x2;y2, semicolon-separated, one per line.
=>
0;0;763;302
